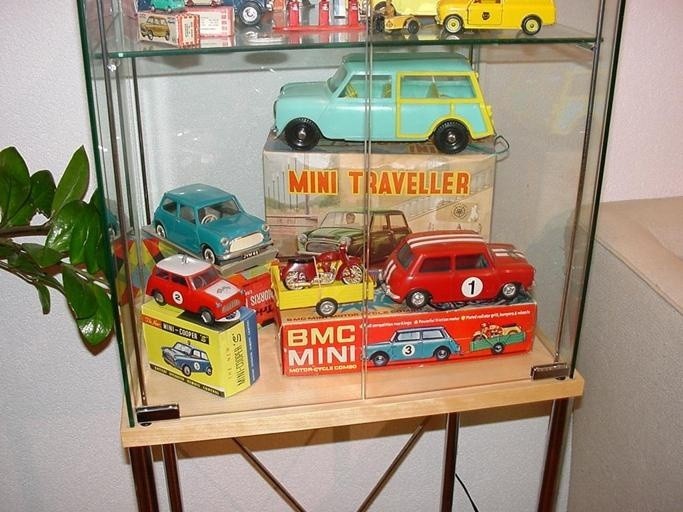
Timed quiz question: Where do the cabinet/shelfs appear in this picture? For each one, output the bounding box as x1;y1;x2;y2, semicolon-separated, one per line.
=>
76;0;627;511
